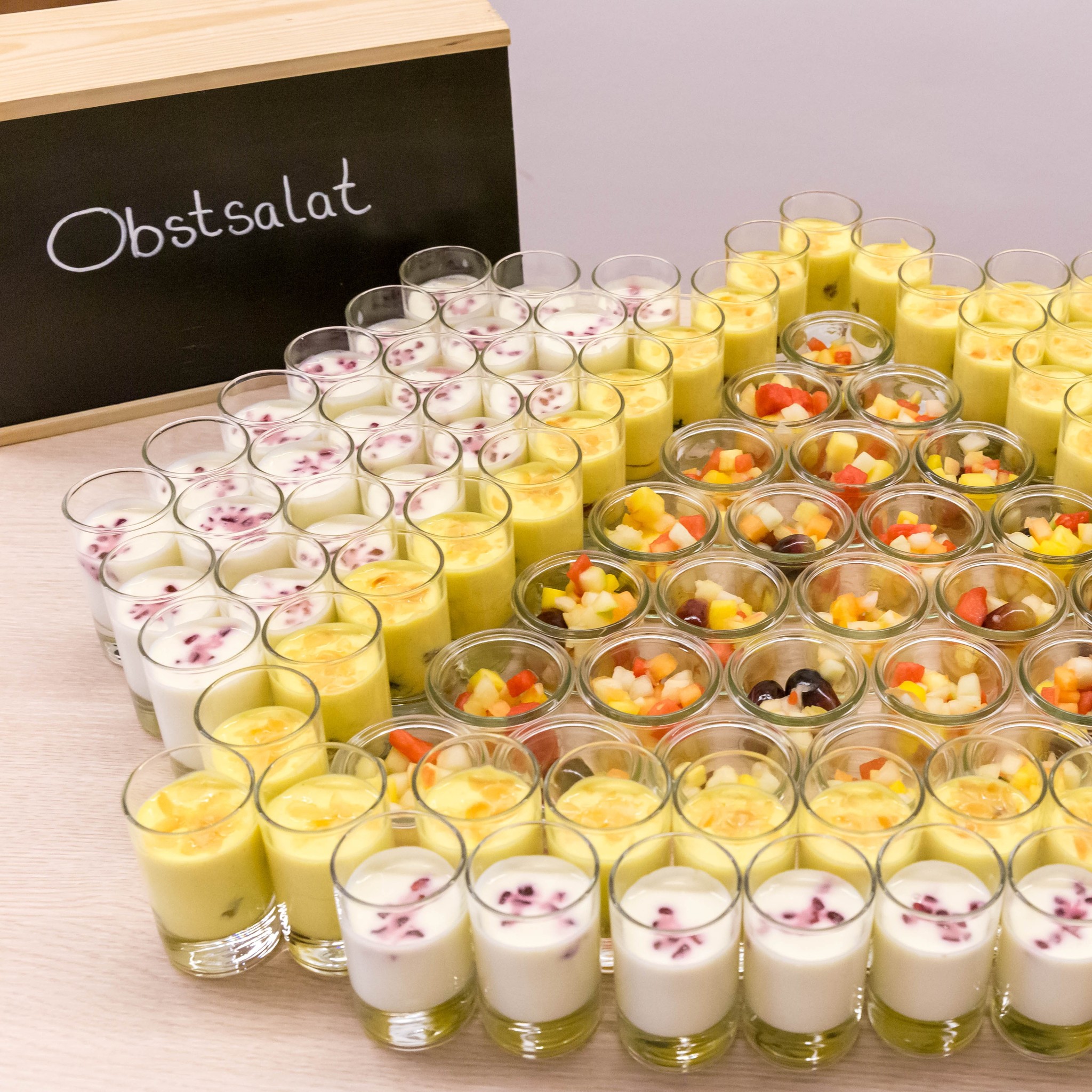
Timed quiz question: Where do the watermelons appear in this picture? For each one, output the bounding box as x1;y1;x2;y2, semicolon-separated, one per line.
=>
390;340;1092;767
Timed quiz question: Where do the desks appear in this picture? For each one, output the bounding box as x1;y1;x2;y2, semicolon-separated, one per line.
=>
0;384;1092;1092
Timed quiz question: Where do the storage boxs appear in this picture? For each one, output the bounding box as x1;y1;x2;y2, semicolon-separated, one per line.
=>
0;0;524;445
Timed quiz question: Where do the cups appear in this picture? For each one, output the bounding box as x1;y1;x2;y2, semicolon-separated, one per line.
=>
59;189;1091;1073
